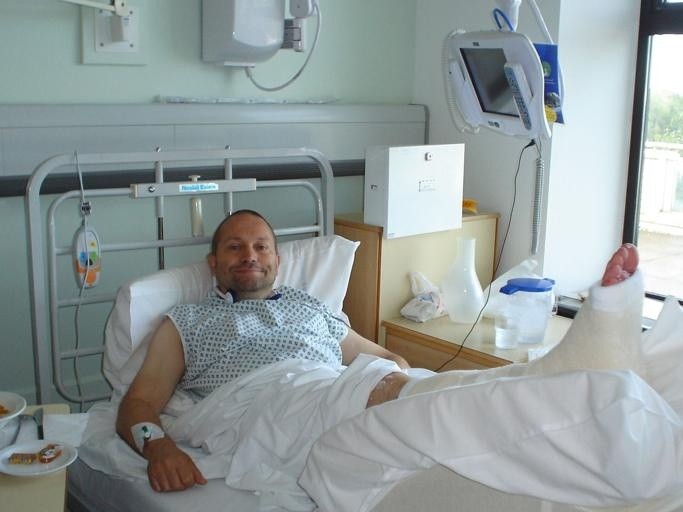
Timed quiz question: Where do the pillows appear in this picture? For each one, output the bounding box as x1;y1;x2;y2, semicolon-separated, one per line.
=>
102;231;360;375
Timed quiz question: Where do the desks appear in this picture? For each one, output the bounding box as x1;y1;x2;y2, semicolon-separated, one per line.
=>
0;402;70;510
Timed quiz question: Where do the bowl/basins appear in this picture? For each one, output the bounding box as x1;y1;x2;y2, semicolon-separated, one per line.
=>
0;390;27;431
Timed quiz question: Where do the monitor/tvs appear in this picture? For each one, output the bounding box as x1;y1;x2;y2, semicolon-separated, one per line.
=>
447;29;551;140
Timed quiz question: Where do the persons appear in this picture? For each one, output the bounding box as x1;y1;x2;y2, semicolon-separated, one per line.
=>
114;207;641;494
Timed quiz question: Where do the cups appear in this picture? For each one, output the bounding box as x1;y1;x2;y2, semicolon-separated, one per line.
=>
494;313;521;351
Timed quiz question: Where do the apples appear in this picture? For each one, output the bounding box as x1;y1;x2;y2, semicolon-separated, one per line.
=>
547;93;560;108
542;61;551;77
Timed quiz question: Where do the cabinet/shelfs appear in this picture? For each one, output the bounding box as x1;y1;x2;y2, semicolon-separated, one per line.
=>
333;210;555;374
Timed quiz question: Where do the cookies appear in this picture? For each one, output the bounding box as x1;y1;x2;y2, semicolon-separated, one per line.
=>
10;445;61;463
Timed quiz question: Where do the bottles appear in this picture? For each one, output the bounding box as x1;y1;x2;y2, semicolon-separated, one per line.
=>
443;237;485;324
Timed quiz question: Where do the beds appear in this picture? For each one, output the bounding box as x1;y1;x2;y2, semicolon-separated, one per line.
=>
22;146;681;511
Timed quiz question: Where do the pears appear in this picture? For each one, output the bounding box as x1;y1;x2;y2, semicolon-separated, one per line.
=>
545;106;556;121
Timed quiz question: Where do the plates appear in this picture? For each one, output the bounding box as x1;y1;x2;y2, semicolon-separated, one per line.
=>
0;439;79;478
